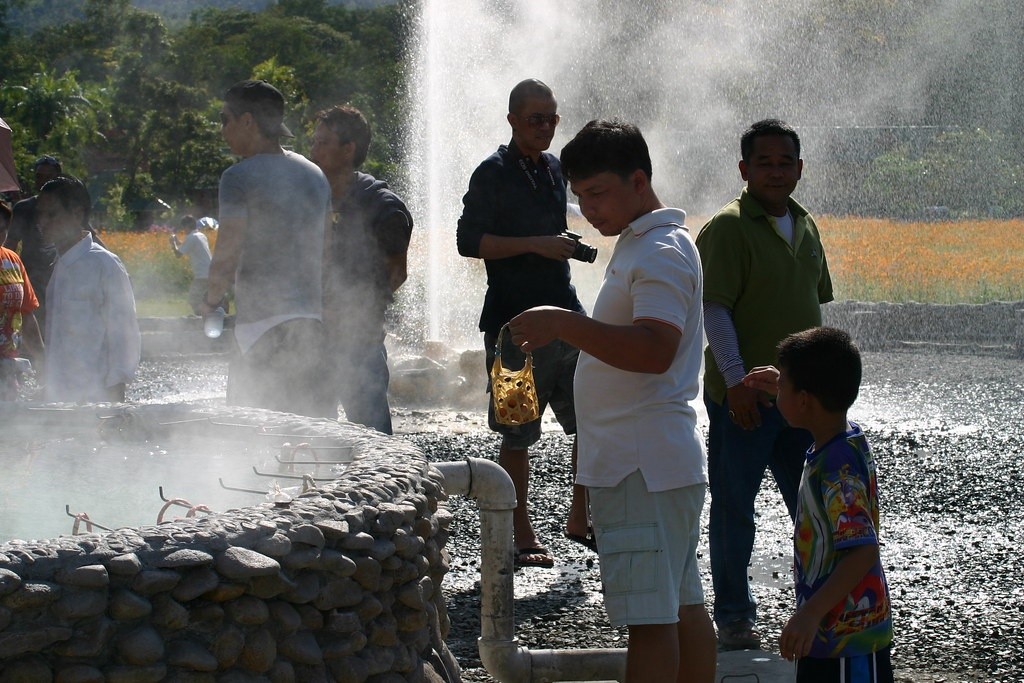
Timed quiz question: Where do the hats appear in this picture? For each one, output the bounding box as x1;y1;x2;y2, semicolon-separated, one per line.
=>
227;79;296;138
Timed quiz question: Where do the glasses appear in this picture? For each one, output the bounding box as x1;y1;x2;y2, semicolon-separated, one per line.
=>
220;112;246;127
512;111;561;127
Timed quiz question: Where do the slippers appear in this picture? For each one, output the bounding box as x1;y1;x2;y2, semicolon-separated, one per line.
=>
563;523;598;547
514;536;554;567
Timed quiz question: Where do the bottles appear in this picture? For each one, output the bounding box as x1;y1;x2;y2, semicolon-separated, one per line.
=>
204;307;224;337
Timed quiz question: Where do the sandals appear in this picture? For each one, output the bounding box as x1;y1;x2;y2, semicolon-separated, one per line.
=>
718;621;763;648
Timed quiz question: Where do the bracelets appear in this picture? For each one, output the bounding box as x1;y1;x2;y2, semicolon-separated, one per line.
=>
202;289;225;308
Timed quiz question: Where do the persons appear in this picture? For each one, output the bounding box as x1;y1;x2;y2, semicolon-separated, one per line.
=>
190;79;332;417
309;105;413;435
143;193;175;232
457;78;598;568
742;326;895;682
508;118;716;683
35;172;142;402
0;193;45;373
4;156;108;343
697;118;835;647
169;216;212;316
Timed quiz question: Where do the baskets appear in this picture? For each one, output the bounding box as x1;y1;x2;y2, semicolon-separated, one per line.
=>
490;321;541;426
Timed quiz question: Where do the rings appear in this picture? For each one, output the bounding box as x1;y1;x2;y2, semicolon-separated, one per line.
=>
728;409;736;419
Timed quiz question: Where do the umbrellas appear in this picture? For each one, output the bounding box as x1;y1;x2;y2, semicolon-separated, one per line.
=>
0;116;23;194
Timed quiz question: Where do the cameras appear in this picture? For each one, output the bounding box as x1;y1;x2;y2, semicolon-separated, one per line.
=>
561;229;597;263
